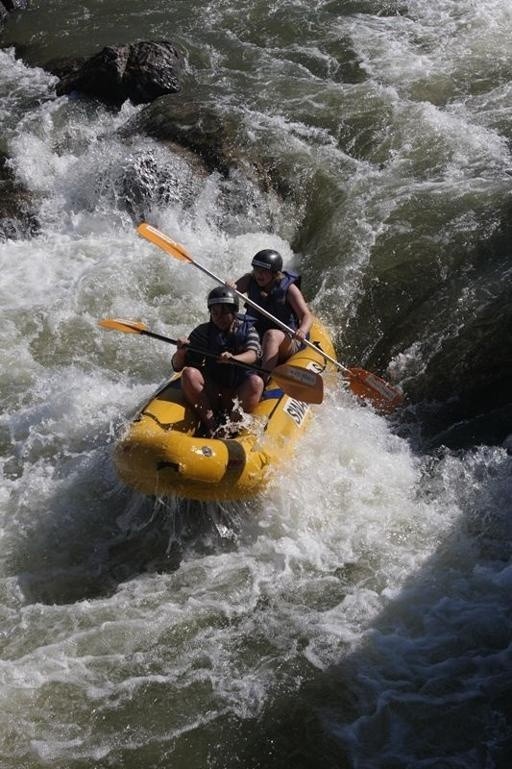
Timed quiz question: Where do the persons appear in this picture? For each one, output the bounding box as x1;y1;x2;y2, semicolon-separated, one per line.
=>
223;248;315;388
170;285;265;441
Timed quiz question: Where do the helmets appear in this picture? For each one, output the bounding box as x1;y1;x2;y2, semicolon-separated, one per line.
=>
208;287;239;312
252;250;282;274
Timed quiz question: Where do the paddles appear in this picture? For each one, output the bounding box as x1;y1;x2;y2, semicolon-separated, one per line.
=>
99;319;323;406
137;224;402;418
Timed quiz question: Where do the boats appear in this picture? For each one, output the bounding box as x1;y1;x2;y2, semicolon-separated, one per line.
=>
109;307;341;508
